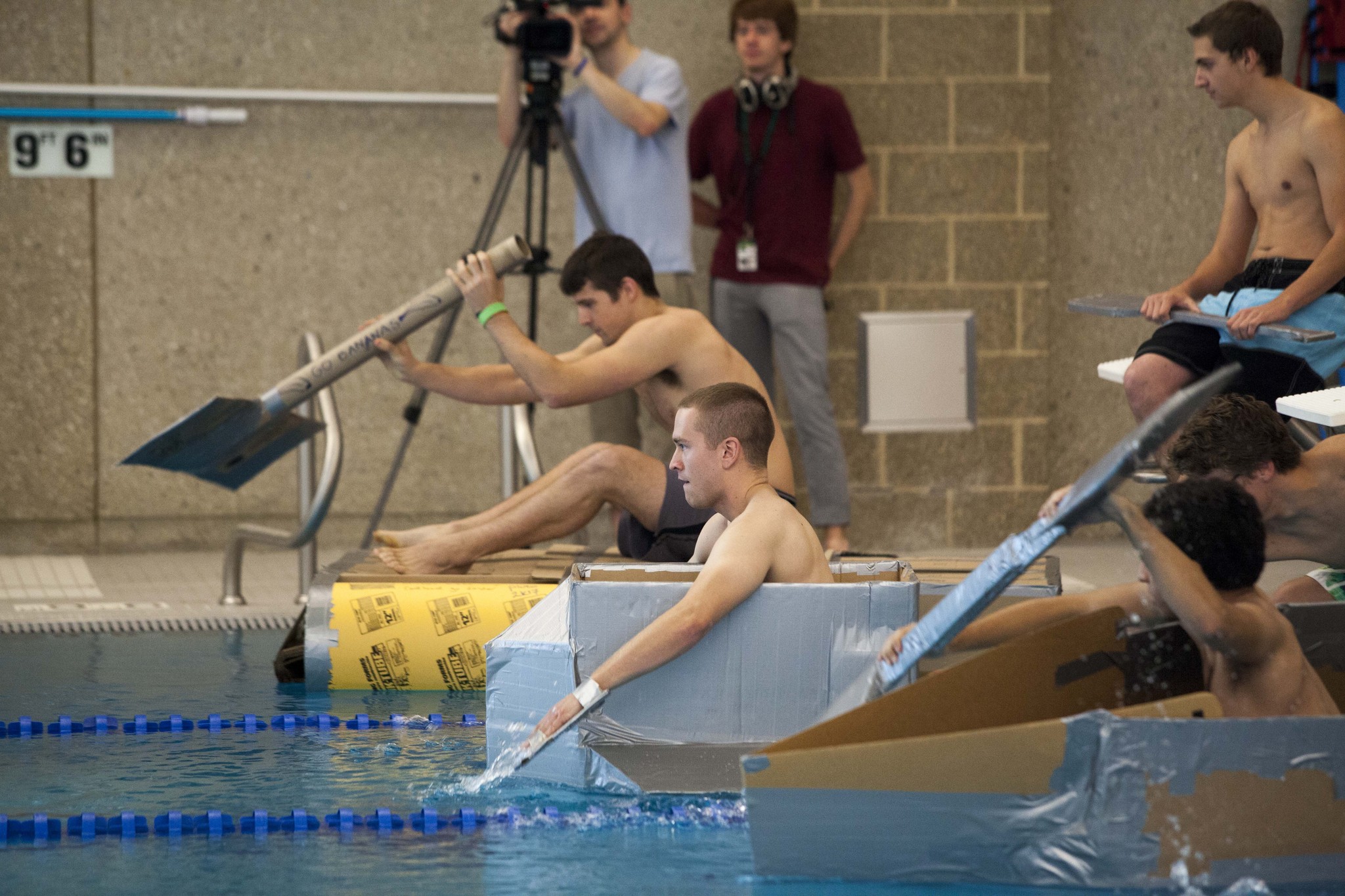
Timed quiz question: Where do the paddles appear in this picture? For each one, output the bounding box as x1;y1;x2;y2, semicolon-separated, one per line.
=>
116;232;533;495
1066;290;1336;344
808;361;1244;730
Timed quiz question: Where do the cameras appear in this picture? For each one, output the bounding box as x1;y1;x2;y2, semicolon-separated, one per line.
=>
492;1;571;59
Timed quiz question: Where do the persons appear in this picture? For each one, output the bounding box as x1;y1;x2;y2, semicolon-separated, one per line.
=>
1123;0;1345;429
684;1;874;551
877;479;1345;720
511;381;836;755
1157;394;1345;603
489;1;710;557
359;235;806;577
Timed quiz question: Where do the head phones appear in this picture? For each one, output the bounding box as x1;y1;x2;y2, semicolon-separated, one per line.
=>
732;64;796;112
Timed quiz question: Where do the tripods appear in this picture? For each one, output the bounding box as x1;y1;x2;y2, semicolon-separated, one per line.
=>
357;61;615;551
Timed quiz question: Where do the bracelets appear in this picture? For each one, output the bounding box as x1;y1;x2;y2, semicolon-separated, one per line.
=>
475;300;509;330
572;53;588;78
